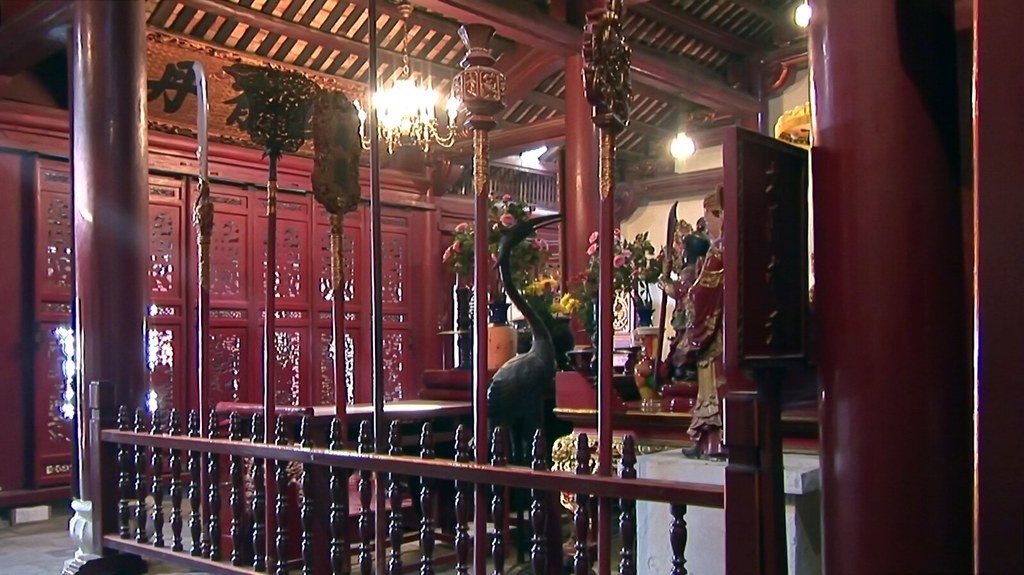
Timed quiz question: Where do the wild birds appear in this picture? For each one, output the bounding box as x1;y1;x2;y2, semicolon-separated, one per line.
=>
482;213;570;566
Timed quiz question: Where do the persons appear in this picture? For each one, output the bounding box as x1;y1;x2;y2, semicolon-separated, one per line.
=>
657;183;724;460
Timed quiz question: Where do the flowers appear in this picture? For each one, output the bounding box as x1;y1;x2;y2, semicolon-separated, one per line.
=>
433;189;664;325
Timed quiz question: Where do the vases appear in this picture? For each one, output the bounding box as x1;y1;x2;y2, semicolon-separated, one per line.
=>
487;300;666;374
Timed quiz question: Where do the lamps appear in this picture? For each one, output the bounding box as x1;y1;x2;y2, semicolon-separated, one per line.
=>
353;0;459;153
